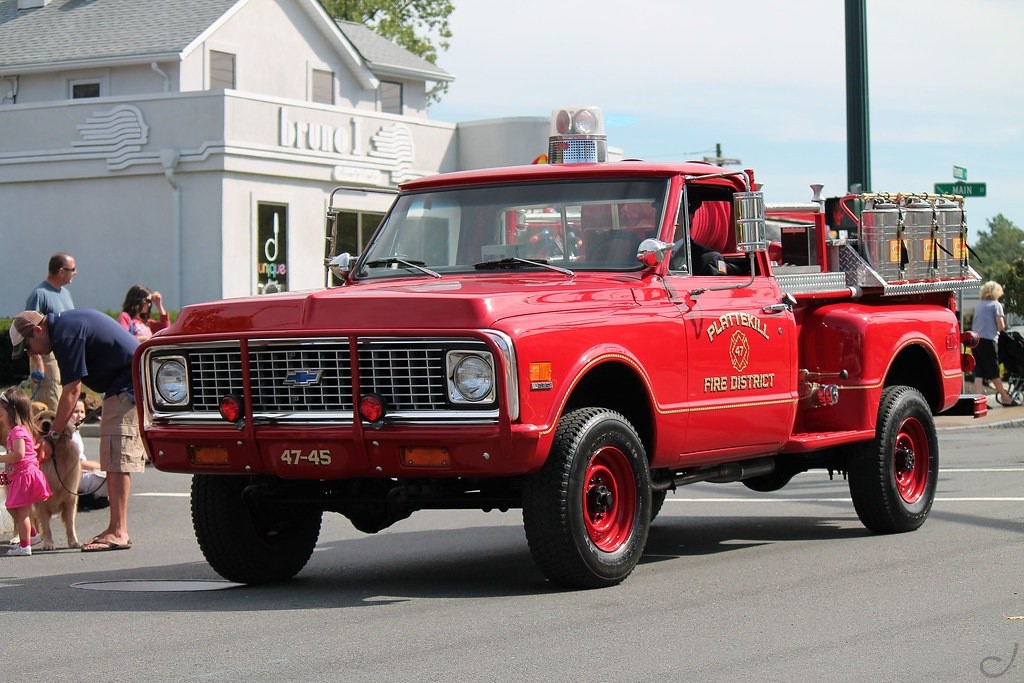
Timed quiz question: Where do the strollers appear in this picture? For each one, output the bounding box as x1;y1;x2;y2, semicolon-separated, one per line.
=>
996;325;1024;405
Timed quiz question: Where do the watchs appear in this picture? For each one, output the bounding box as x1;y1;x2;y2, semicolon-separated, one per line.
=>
48;430;60;439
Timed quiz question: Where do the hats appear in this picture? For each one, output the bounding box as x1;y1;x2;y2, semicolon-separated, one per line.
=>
9;311;44;358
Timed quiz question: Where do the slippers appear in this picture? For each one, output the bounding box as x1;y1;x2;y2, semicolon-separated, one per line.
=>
1002;400;1022;407
987;405;992;409
81;534;132;552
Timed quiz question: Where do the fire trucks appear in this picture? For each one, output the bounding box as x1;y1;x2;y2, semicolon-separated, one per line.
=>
136;162;981;588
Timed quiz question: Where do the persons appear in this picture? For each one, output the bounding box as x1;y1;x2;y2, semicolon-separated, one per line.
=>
116;285;169;343
9;308;146;552
971;280;1018;408
71;392;108;498
593;192;738;275
0;386;53;556
26;253;77;413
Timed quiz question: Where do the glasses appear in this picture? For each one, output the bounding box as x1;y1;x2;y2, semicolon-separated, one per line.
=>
144;299;151;303
0;392;9;403
63;267;75;271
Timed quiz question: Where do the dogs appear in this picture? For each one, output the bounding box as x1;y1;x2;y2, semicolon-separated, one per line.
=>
29;410;83;550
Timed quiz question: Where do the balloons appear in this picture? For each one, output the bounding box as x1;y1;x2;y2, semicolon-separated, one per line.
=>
546;208;553;213
569;232;575;239
542;228;550;235
577;240;583;247
529;236;539;243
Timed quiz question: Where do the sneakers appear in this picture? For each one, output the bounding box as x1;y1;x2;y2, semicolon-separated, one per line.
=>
13;534;41;548
6;545;31;555
